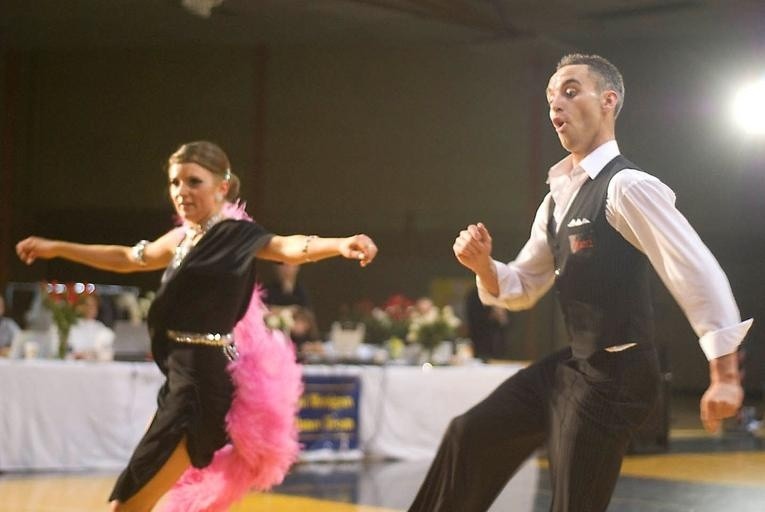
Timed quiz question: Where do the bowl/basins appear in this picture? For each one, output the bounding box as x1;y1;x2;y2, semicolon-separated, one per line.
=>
181;212;222;240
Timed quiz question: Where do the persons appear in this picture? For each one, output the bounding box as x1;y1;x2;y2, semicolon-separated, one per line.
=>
64;295;111;363
722;391;765;450
410;52;757;510
259;255;315;350
16;140;379;512
288;304;313;348
467;281;510;360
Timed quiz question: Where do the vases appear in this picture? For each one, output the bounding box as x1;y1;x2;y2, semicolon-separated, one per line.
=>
373;292;458;363
38;278;96;357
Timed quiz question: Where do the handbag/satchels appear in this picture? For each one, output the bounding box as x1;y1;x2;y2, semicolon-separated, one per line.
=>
302;234;322;264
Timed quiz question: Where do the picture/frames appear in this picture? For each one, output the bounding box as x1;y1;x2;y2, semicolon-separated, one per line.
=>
1;353;530;477
